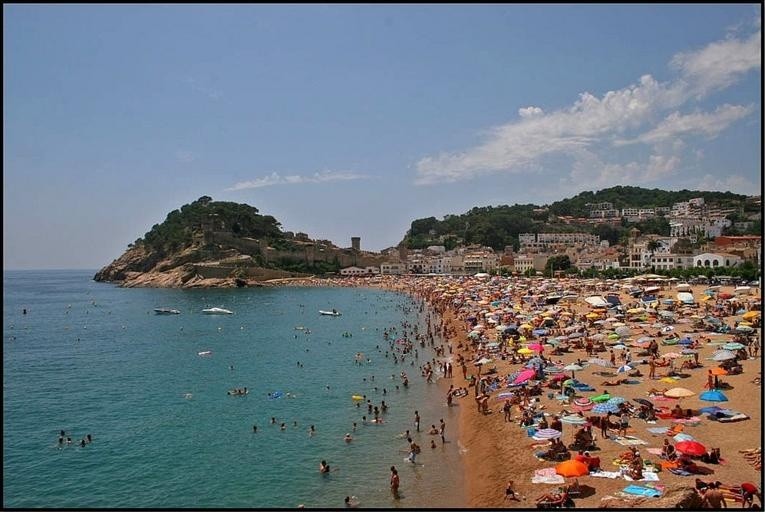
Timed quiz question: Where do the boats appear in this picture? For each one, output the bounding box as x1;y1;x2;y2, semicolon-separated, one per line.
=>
319;310;340;316
202;306;234;315
154;309;181;315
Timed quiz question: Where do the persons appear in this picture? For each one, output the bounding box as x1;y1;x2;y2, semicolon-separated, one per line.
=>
311;273;762;508
57;430;91;448
227;386;248;397
229;365;236;371
253;417;299;435
